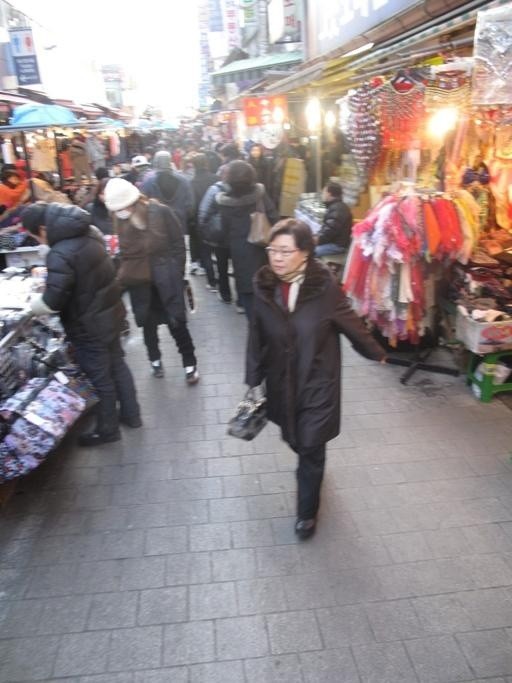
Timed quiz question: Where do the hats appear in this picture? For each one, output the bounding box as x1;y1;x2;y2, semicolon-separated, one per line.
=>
103;178;141;212
154;151;171;171
132;156;151;168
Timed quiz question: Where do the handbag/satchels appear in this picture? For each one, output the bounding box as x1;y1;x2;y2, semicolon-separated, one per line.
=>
247;212;271;247
208;212;226;242
227;386;269;441
182;281;196;314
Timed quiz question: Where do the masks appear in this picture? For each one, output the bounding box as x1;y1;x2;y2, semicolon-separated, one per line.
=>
115;210;131;221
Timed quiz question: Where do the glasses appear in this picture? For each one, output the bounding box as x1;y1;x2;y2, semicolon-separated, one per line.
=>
265;247;302;257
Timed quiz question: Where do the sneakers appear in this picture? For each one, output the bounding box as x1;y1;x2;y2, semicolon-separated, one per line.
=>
206;284;218;293
120;412;142;429
191;263;198;273
217;292;231;304
185;366;198;383
150;361;164;378
198;268;207;276
235;302;245;314
79;429;121;446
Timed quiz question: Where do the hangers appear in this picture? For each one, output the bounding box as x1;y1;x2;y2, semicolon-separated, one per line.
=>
335;56;483;104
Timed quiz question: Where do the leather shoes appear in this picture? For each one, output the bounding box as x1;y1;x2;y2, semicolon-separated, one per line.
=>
295;516;316;536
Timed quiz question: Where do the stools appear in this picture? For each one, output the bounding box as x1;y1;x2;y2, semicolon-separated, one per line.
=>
467;352;512;404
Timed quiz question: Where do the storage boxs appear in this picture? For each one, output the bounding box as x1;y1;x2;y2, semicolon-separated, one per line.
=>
454;308;512;356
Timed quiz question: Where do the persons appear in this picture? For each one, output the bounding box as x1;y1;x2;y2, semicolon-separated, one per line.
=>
78;165;118;236
245;144;276;202
131;130;230;153
73;131;96;171
20;200;143;446
19;178;75;206
106;131;128;166
102;177;200;384
199;164;232;293
189;154;226;289
273;141;309;222
85;134;108;171
225;216;387;540
217;144;245;179
140;149;198;315
0;169;28;221
113;162;140;186
130;154;155;192
179;151;207;177
70;136;88;176
214;160;278;316
59;142;72;177
309;178;352;290
462;153;490;187
322;129;348;188
